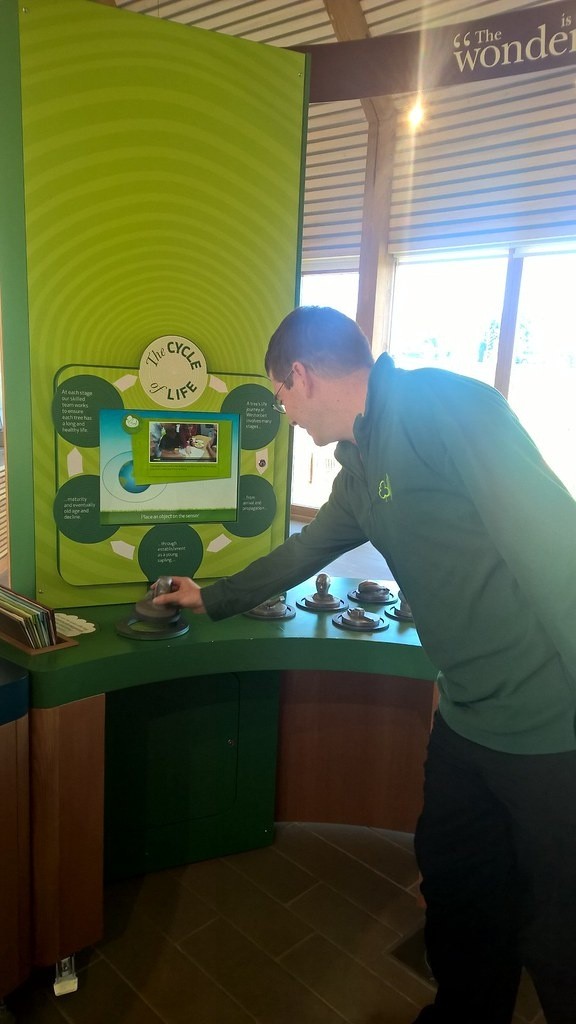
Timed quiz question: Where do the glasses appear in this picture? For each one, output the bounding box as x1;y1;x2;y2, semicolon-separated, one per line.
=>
271;367;299;415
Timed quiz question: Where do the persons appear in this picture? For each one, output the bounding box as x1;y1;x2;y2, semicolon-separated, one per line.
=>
150;423;217;462
150;305;576;1024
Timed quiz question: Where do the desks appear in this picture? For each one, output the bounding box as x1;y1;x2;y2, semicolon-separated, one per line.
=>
0;578;440;1000
